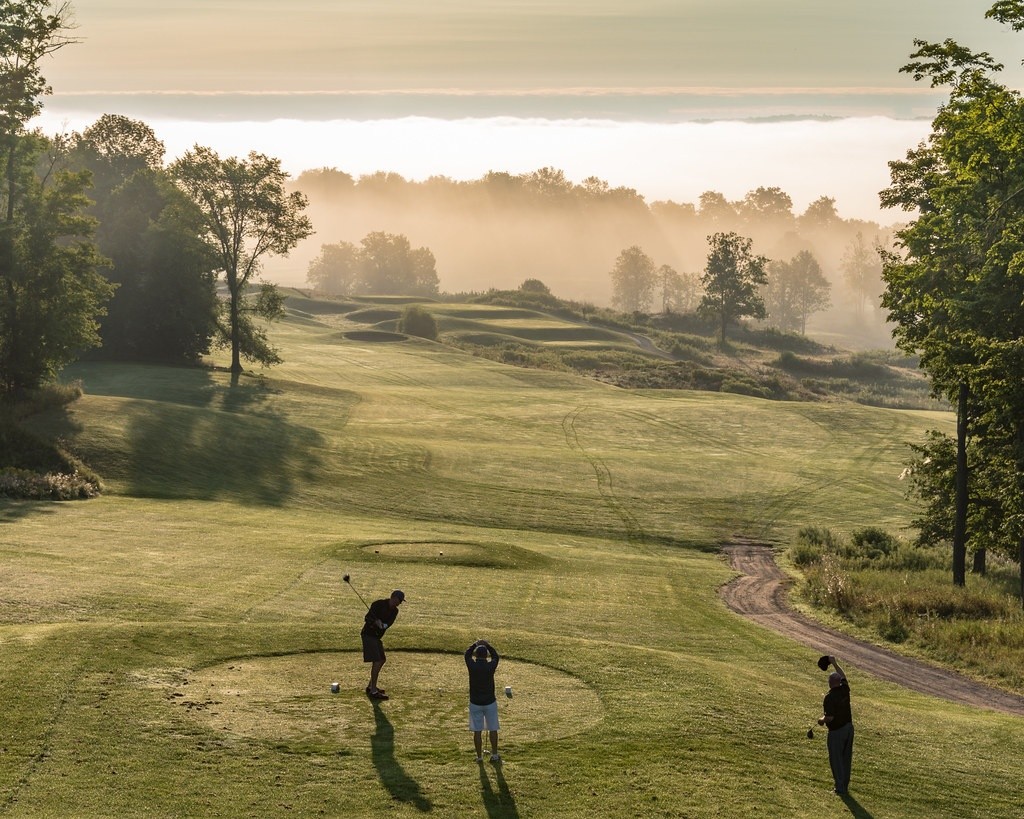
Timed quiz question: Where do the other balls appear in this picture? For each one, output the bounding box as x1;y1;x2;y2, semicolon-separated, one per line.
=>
331;683;339;691
505;687;511;694
440;552;443;555
375;551;379;553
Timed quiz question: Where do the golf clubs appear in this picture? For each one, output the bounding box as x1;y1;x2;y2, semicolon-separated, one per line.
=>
483;731;491;754
343;573;378;621
807;723;818;739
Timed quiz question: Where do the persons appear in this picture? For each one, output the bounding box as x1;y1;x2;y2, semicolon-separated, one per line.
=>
817;655;854;792
360;590;406;700
465;639;500;762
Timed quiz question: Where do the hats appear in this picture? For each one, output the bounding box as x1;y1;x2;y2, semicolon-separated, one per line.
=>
818;656;830;672
476;645;487;654
392;589;406;601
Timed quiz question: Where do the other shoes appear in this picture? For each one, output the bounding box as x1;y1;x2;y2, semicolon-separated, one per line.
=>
835;789;848;796
476;759;483;763
489;757;501;763
369;692;389;700
365;687;385;693
